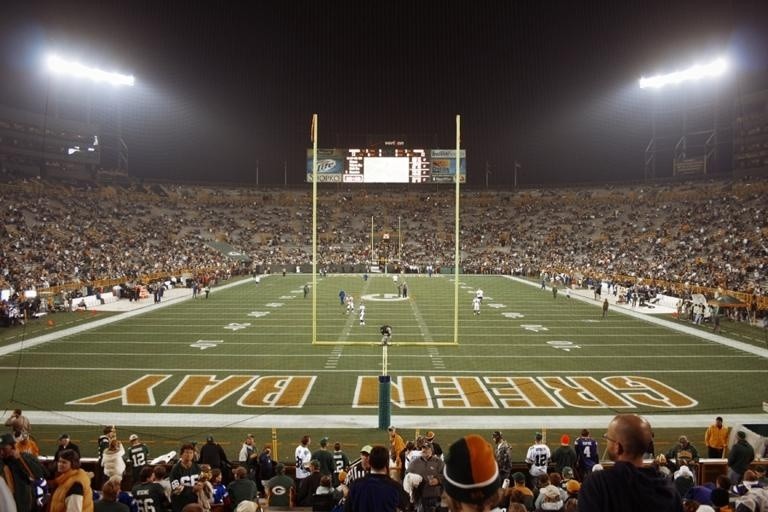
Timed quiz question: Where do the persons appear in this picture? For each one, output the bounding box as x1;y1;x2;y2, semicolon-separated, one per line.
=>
378;323;394;346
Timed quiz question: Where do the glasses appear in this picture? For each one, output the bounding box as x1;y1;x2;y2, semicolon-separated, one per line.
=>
600;432;617;445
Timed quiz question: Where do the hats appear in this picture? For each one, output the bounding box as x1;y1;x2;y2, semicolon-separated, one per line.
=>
424;431;435;439
320;436;329;445
358;444;373;455
510;472;527;482
539;483;562;500
441;432;502;505
58;432;71;441
563;479;582;494
535;432;574;481
129;434;138;441
